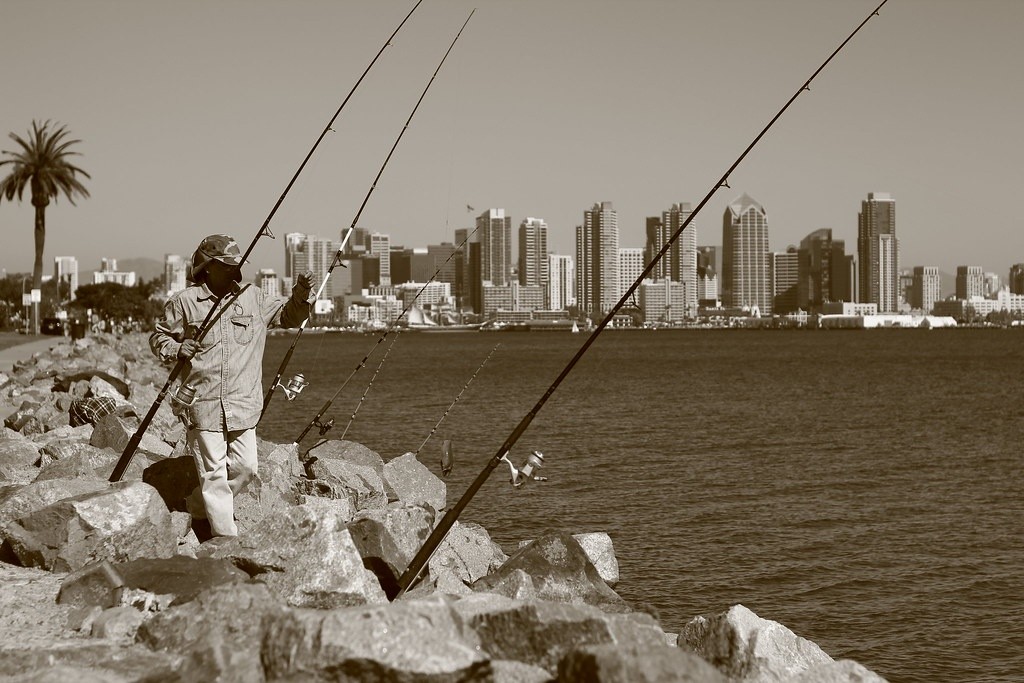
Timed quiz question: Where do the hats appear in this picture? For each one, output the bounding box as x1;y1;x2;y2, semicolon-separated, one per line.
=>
191;235;249;285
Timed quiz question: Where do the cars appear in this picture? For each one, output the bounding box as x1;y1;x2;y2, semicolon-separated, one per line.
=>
42;318;63;336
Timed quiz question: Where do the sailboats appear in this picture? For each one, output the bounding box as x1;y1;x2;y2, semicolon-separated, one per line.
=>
571;321;580;334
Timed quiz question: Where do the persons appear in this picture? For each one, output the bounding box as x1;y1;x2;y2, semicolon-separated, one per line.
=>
150;233;316;538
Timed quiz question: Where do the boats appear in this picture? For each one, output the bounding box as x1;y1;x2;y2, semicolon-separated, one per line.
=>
478;321;531;332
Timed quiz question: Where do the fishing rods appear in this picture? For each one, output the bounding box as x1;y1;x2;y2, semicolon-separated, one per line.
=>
107;0;889;604
